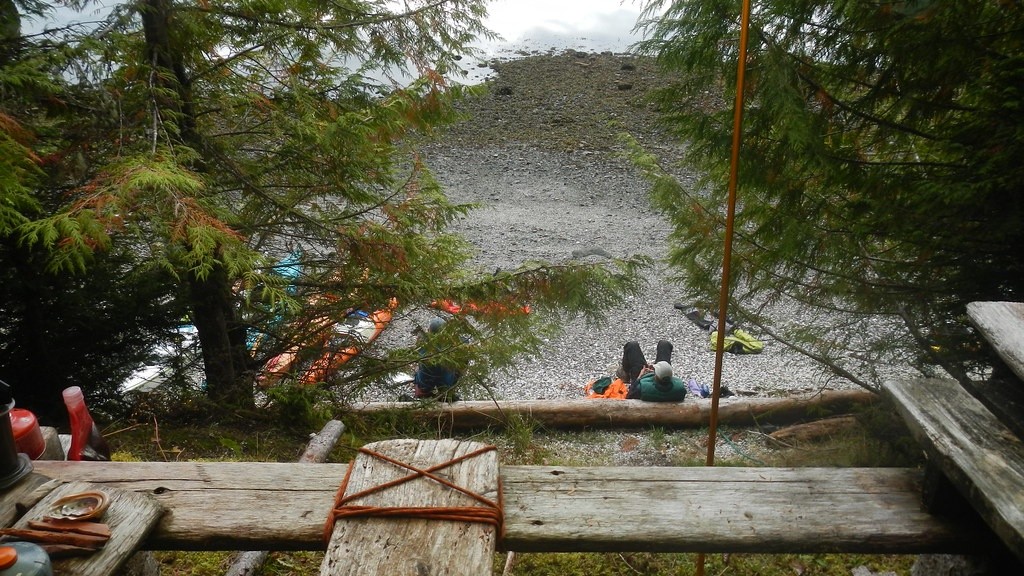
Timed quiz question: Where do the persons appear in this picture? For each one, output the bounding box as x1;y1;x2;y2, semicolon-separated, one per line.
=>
414;319;469;402
616;339;687;402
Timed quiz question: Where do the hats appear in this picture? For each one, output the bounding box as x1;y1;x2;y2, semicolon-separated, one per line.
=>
429;318;445;333
653;361;672;379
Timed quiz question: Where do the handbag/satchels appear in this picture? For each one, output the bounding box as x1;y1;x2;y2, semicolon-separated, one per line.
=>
584;379;628;400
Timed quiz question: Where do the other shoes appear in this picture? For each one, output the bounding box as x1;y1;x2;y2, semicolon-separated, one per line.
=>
617;367;630;383
